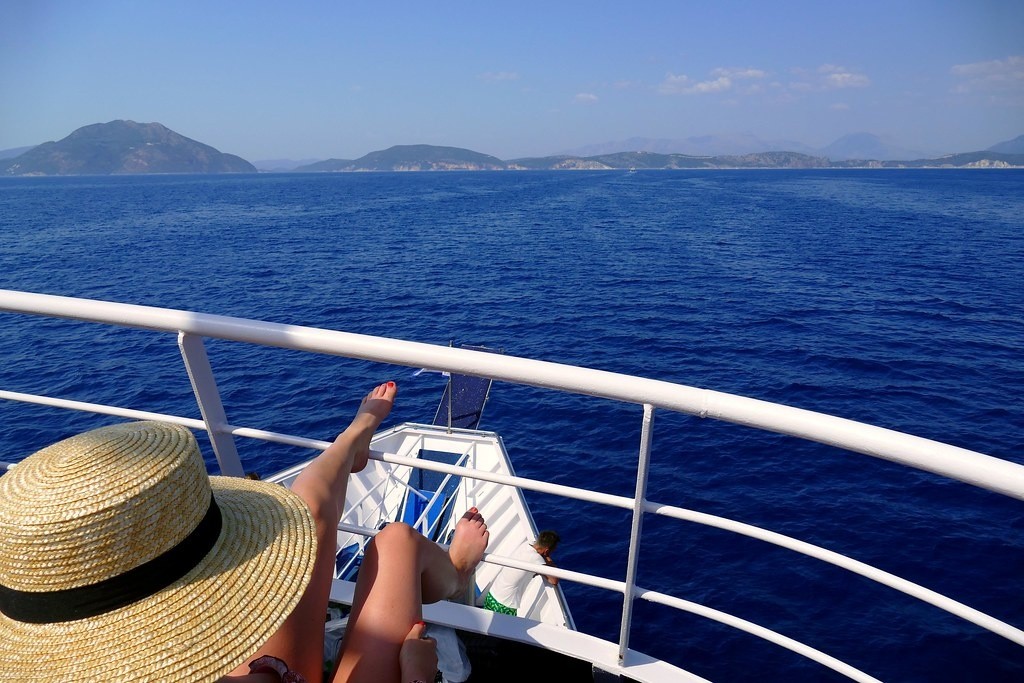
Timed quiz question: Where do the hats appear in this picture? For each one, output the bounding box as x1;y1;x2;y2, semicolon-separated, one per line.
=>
0;422;317;683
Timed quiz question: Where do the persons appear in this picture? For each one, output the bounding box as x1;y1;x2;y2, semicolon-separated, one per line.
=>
482;527;563;618
0;375;491;682
